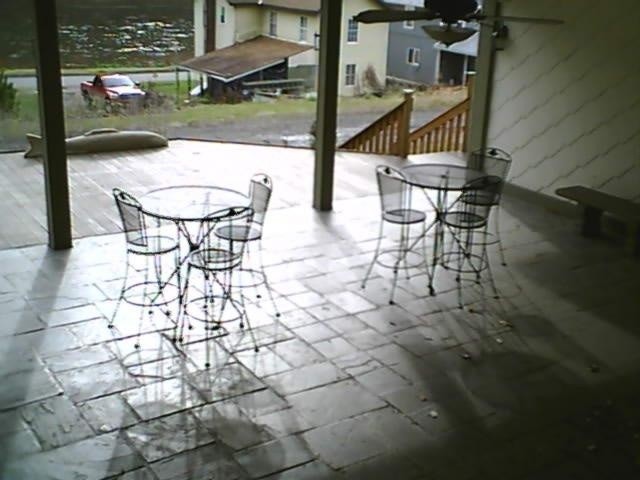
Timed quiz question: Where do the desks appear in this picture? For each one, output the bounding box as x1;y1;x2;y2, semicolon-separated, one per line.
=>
133;183;255;346
393;162;488;296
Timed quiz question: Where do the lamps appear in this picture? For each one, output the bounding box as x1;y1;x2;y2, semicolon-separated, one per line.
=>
421;25;479;47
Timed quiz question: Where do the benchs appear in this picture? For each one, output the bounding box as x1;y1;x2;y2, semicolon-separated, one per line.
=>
555;185;640;239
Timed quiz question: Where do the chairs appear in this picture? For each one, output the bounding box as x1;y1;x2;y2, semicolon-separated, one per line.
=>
358;162;436;305
180;205;261;367
428;173;501;311
206;171;283;322
109;186;194;350
458;149;510;284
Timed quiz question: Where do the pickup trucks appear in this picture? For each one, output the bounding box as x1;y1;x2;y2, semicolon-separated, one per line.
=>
79;70;148;113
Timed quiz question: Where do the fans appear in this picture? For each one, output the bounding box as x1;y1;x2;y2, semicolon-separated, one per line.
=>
352;0;570;25
